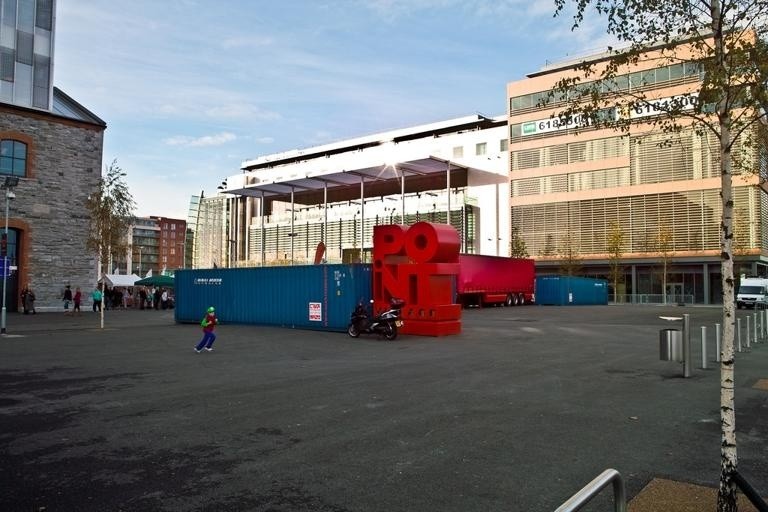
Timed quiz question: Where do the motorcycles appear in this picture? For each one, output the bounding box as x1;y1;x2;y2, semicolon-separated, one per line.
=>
347;298;405;340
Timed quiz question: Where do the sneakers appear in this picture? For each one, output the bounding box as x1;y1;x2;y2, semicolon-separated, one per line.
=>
205;348;213;351
194;348;201;353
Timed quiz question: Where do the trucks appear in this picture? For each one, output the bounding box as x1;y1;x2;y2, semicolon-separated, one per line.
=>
456;254;534;307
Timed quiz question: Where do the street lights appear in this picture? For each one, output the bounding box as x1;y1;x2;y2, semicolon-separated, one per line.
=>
0;188;15;334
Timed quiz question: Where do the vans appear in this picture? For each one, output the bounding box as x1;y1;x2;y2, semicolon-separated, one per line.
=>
736;278;768;309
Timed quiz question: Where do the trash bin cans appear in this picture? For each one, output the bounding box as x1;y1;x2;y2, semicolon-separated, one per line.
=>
659;316;685;362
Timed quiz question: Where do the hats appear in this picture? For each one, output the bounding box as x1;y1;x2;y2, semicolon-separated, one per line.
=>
207;307;215;313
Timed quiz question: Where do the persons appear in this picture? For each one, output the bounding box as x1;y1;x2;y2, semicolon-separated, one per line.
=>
71;287;82;317
92;283;175;313
61;285;72;316
193;307;218;354
20;283;36;316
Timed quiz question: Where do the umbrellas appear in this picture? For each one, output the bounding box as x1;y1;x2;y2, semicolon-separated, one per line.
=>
133;274;174;308
